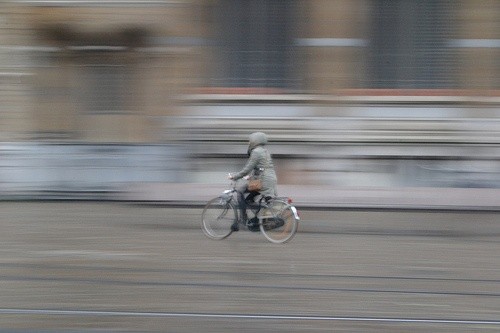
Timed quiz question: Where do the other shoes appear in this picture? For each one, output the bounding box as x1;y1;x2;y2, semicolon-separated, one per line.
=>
230;220;248;230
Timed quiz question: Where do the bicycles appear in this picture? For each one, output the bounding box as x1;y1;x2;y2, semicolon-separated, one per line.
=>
200;173;299;244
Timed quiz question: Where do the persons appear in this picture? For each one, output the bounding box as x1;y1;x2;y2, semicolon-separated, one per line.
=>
229;132;277;222
